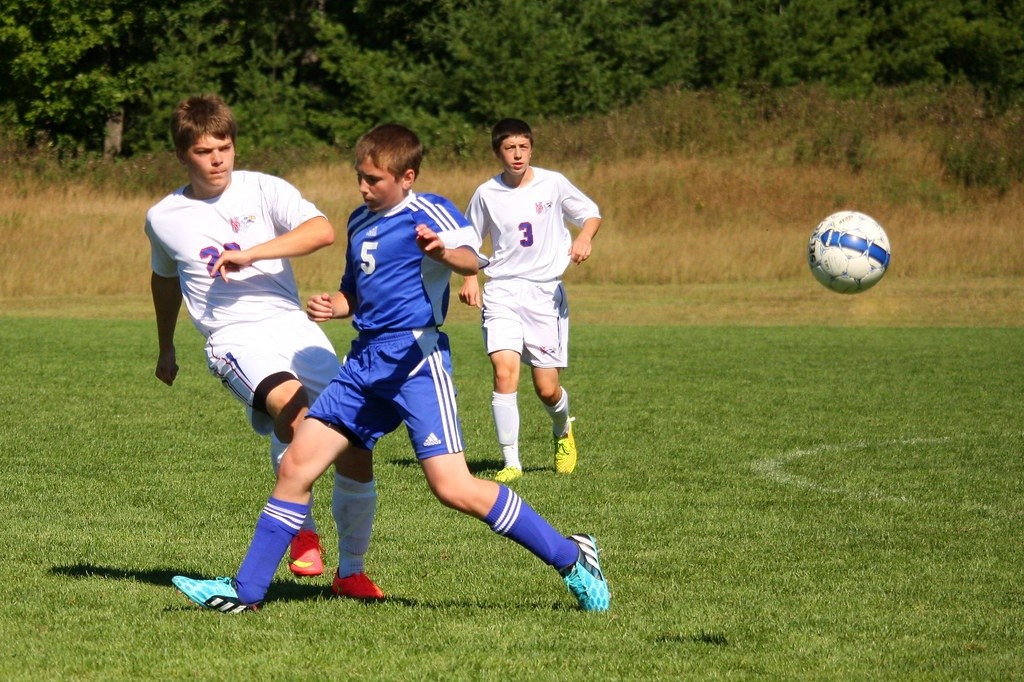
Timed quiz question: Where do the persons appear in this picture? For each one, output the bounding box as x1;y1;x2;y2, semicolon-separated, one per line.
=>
458;118;603;483
144;91;386;603
171;122;611;615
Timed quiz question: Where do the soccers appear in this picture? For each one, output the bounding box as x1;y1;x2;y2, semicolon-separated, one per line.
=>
807;208;890;294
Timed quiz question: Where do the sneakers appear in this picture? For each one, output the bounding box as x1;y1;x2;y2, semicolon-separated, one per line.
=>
494;466;523;483
289;528;326;577
171;575;247;616
553;416;577;476
332;566;384;599
559;533;608;613
